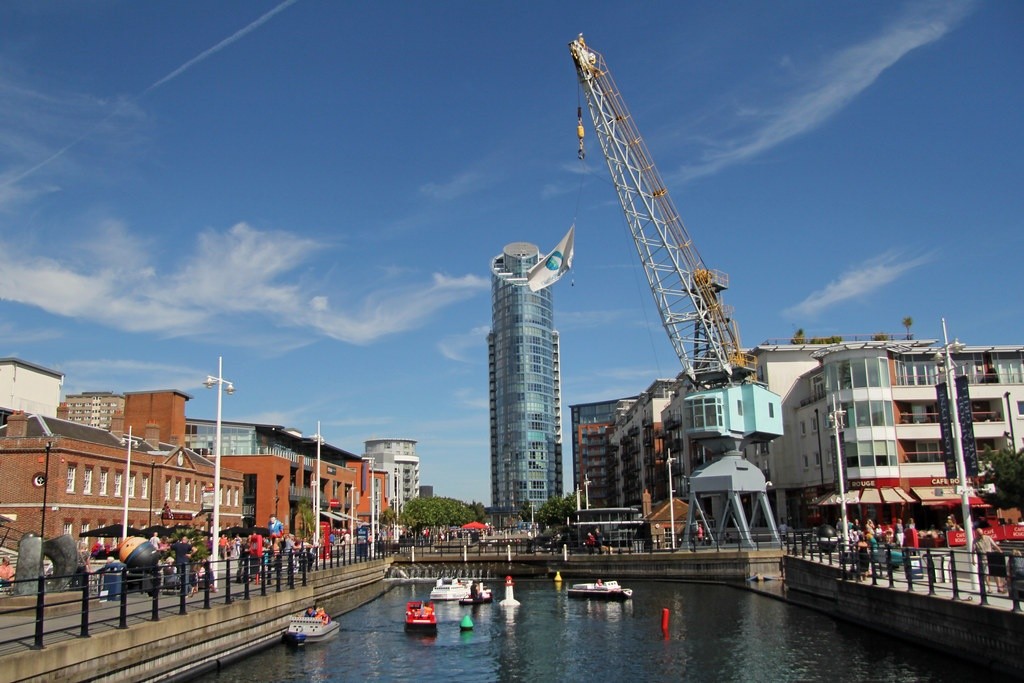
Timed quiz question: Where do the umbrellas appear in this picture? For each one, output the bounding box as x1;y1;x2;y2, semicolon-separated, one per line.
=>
462;521;488;532
221;525;269;538
78;523;211;548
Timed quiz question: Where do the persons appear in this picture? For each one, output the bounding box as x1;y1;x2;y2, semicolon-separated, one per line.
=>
77;542;114;573
595;579;602;588
150;532;219;597
779;521;788;543
836;516;916;583
584;528;605;555
0;556;15;588
329;522;539;550
945;513;1024;595
697;524;704;545
422;602;432;615
304;607;331;627
219;533;322;574
267;514;284;537
470;579;477;598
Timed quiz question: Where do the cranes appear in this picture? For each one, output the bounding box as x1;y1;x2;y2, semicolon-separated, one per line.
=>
568;33;786;552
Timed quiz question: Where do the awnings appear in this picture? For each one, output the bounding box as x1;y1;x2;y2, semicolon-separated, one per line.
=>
809;485;982;508
320;511;362;522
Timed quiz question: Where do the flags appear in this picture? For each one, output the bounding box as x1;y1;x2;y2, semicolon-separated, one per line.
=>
164;501;174;519
527;222;573;292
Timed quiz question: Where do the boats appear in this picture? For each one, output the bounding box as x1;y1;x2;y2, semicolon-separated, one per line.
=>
566;580;633;601
405;598;437;630
283;613;342;647
429;576;493;606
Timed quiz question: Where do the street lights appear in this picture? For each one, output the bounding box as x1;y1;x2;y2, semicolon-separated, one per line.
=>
584;480;592;509
202;357;236;593
828;408;851;552
311;420;326;544
361;456;376;561
119;425;139;541
528;504;536;525
666;447;677;550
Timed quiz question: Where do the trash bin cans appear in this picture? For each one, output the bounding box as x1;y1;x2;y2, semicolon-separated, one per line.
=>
902;548;924;579
359;543;368;557
103;562;127;601
